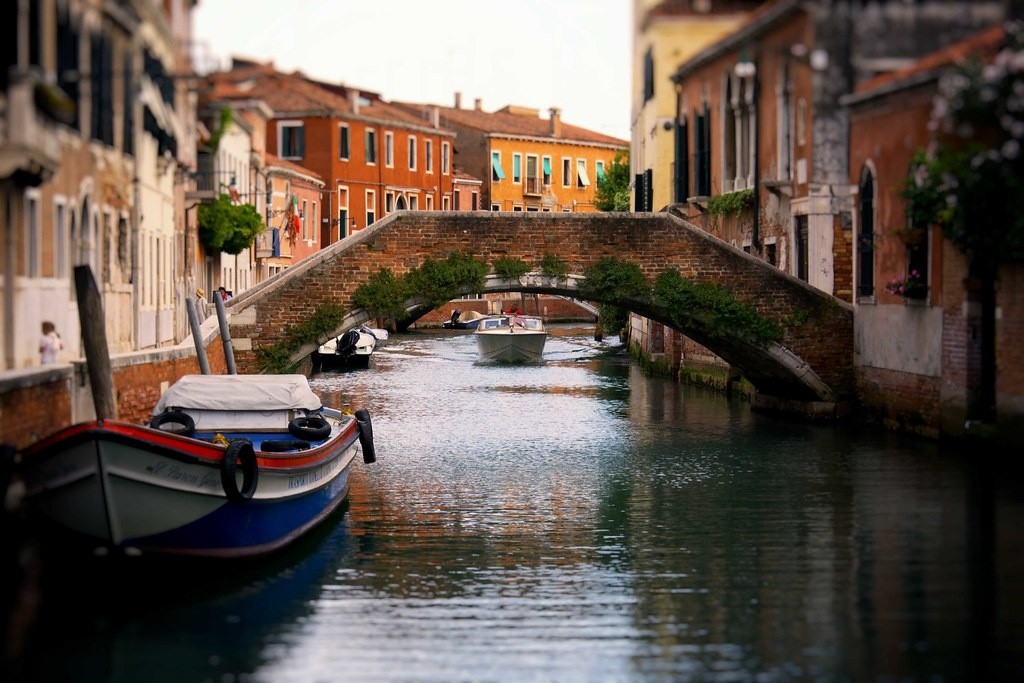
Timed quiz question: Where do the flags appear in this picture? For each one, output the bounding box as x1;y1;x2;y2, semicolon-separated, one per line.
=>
284;193;301;248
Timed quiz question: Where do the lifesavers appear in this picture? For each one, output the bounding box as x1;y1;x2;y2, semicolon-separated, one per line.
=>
223;438;259;503
150;411;195;436
288;416;332;441
355;409;377;464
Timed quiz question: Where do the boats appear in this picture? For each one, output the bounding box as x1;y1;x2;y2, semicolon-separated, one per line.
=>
357;326;388;347
19;272;377;592
443;307;494;328
318;322;375;369
472;310;549;369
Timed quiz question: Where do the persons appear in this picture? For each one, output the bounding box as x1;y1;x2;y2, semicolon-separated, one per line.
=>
196;288;208;326
39;322;64;365
506;302;523;315
219;287;230;302
